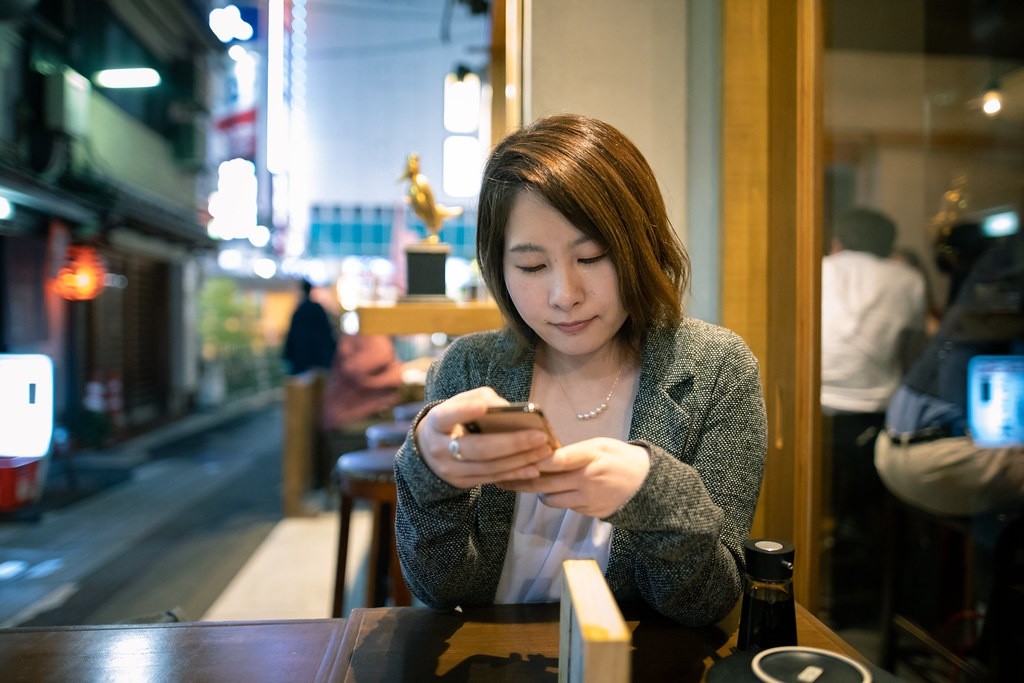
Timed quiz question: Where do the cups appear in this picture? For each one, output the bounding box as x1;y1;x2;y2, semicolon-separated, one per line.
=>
738;537;798;656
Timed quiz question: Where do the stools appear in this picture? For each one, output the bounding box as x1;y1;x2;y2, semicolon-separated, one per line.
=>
878;494;978;674
331;447;413;617
367;421;410;608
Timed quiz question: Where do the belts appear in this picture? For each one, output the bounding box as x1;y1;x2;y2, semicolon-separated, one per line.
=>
884;426;967;445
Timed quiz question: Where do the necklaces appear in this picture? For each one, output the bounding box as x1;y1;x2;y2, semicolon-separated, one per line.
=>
549;350;631;421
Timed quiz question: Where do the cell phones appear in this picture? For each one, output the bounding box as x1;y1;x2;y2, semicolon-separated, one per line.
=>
459;404;562;491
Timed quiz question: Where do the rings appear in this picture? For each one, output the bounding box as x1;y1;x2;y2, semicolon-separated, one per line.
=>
449;435;463;461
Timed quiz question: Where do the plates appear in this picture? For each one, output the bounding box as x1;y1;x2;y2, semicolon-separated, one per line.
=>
705;646;875;683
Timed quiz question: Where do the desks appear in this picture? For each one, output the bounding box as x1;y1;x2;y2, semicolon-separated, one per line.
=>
329;603;861;683
0;618;349;683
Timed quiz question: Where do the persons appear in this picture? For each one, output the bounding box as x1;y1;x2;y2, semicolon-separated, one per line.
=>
872;227;1024;516
392;113;767;627
823;210;929;630
933;218;994;307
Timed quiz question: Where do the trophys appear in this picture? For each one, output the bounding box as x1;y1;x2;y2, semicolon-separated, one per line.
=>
395;152;462;304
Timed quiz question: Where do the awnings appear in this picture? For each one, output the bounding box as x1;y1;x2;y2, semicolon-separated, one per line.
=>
93;183;219;256
0;162;95;226
